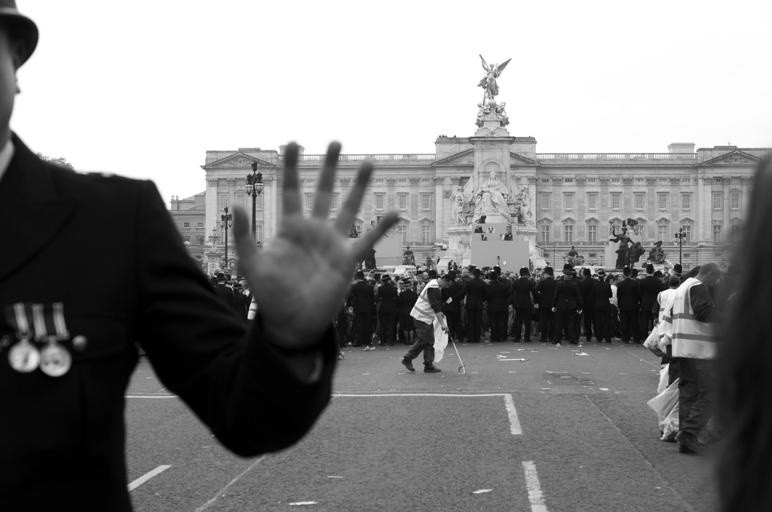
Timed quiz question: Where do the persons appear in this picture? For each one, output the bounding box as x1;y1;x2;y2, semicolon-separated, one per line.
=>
403;246;414;261
478;60;498;99
708;152;772;512
0;0;400;512
610;227;635;266
649;241;666;263
562;246;578;260
450;170;533;225
670;263;725;453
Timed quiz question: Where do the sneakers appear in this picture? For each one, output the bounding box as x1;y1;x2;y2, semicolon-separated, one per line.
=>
679;442;696;453
678;431;707;456
424;366;442;373
401;357;415;372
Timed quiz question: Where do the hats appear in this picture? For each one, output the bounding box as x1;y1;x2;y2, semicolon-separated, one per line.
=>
542;266;554;276
622;265;631;275
0;0;39;71
646;264;654;274
630;269;639;278
654;271;664;278
456;265;502;280
415;263;438;278
583;268;591;277
597;269;605;277
520;268;530;277
672;264;682;273
354;269;364;280
561;264;577;275
374;273;412;284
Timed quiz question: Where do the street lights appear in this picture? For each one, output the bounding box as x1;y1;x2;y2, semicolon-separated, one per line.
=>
208;226;222;253
674;226;686;272
220;207;233;268
246;161;264;244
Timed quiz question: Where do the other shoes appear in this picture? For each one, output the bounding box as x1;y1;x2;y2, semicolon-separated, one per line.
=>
623;335;647;344
585;332;612;343
490;335;532;343
447;334;481;343
540;335;579;344
339;337;413;345
